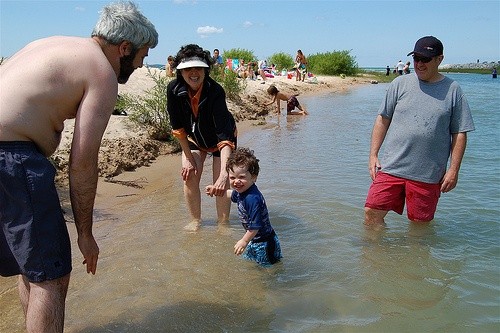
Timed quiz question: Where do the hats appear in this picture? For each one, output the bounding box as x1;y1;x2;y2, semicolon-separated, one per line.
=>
407;36;443;58
176;55;209;69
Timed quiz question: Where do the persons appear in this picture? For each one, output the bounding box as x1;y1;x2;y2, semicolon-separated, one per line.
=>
167;48;308;83
0;0;159;333
205;146;283;267
385;58;410;75
262;85;311;116
167;44;238;235
363;36;476;227
492;66;497;78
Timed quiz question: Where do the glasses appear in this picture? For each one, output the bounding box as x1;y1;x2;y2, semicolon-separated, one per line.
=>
412;55;439;63
